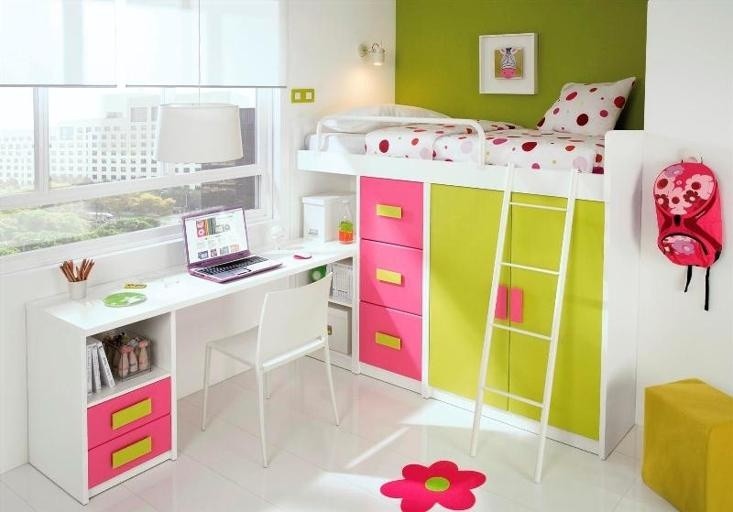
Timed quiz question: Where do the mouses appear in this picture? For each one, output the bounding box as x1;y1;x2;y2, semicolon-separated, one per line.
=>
293;253;312;260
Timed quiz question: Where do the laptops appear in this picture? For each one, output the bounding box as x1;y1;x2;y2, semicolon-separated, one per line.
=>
181;204;282;282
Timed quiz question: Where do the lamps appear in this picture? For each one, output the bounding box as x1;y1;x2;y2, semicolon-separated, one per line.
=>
358;41;385;66
154;2;246;167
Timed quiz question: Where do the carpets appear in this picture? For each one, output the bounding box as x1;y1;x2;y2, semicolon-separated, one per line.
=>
378;458;487;512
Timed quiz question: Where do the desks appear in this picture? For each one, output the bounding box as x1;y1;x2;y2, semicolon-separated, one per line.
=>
25;239;357;507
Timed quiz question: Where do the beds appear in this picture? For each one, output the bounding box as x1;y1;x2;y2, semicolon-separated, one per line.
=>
295;107;639;461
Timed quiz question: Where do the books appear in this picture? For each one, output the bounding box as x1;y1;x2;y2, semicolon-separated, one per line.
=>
86;336;116;396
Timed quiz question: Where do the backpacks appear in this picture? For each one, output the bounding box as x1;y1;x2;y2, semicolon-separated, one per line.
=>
653;152;723;266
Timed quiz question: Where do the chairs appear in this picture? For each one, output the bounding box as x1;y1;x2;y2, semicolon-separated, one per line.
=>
200;270;341;470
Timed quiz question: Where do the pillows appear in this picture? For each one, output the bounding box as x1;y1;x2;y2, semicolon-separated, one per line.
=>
535;76;638;138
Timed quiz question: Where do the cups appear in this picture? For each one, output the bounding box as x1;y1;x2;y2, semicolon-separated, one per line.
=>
67;277;88;301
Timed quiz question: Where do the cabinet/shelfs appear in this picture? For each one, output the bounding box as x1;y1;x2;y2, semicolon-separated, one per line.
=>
293;156;427;397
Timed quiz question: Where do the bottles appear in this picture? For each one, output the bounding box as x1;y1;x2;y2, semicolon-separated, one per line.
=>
337;198;354;245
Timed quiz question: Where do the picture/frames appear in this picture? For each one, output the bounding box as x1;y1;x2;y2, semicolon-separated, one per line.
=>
478;32;538;96
